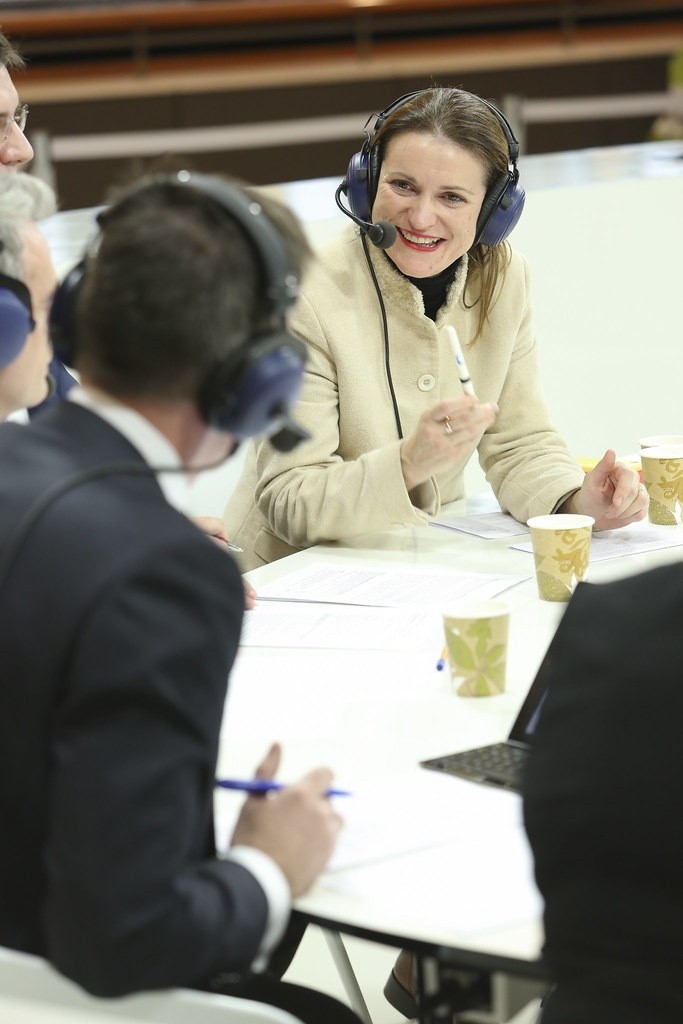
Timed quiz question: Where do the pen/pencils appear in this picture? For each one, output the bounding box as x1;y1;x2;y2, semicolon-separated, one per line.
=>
213;777;347;797
216;536;245;552
447;327;478;401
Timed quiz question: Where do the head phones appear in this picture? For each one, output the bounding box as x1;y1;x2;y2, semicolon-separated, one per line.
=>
0;241;37;372
47;174;310;437
335;85;526;249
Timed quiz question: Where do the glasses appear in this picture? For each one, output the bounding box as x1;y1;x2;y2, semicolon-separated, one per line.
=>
0;104;29;144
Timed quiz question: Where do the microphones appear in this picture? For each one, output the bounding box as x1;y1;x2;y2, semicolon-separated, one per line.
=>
268;402;309;451
335;179;397;250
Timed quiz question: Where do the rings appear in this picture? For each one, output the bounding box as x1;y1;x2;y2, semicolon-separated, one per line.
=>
446;420;454;434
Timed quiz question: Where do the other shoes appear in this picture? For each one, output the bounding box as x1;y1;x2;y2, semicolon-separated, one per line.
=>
384;948;489;1020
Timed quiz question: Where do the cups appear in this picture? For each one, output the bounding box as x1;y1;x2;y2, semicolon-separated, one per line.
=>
526;514;595;602
638;434;683;452
441;602;511;697
639;446;683;525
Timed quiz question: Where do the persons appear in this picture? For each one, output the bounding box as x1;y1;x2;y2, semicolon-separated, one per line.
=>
0;33;64;426
0;166;367;1023
519;561;683;1024
219;89;651;1024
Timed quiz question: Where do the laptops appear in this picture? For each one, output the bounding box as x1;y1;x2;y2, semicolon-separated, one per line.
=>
420;581;594;794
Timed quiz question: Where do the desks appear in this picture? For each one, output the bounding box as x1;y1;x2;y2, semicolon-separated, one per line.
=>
205;485;683;1024
